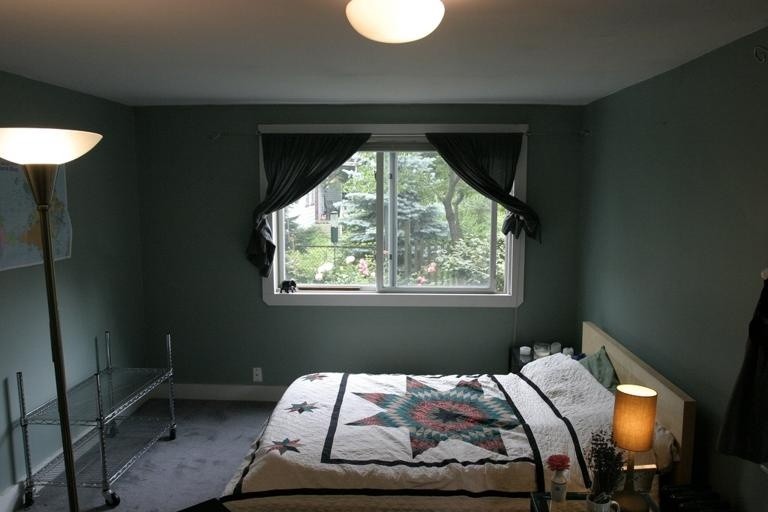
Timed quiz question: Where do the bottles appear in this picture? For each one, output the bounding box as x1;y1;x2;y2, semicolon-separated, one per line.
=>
563;346;574;356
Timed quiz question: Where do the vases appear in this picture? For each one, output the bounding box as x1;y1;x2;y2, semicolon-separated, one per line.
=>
550;469;567;512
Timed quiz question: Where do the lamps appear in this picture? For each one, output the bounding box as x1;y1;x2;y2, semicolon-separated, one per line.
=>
0;127;103;512
341;1;445;46
611;383;657;511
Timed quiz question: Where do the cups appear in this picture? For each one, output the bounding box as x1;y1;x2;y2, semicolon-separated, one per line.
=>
551;342;562;354
586;494;620;512
520;346;531;355
533;343;549;357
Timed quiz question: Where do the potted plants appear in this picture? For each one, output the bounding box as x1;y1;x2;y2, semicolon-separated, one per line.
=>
582;426;626;512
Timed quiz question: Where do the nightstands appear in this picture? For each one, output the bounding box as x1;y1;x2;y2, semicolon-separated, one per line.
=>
508;342;577;374
530;492;659;512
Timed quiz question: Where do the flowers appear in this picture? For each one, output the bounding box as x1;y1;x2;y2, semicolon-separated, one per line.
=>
547;454;570;472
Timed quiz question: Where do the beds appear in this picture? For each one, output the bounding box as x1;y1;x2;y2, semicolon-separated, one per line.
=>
219;321;695;511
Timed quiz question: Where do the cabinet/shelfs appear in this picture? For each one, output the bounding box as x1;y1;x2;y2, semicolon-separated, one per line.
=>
25;367;173;489
